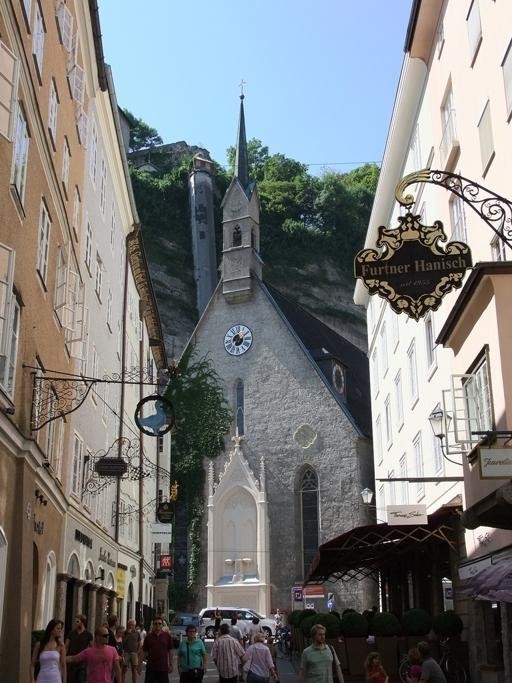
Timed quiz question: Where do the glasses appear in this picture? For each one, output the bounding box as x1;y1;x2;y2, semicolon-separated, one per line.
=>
153;623;162;625
102;633;110;638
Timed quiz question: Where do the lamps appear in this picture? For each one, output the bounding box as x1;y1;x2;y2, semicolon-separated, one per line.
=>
428;402;463;466
360;487;387;523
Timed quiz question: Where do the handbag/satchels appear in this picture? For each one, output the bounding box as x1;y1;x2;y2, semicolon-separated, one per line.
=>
328;644;339;683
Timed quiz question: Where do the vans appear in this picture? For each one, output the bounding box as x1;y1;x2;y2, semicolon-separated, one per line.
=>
198;606;277;639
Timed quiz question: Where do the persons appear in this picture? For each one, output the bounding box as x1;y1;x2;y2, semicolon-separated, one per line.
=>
298;624;344;682
177;624;208;682
31;614;172;682
406;641;448;682
365;652;389;682
210;608;283;682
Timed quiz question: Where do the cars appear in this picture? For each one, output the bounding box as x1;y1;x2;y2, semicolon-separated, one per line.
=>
168;612;206;645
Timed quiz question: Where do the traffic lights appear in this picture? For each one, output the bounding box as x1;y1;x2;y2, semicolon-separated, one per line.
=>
160;554;173;569
